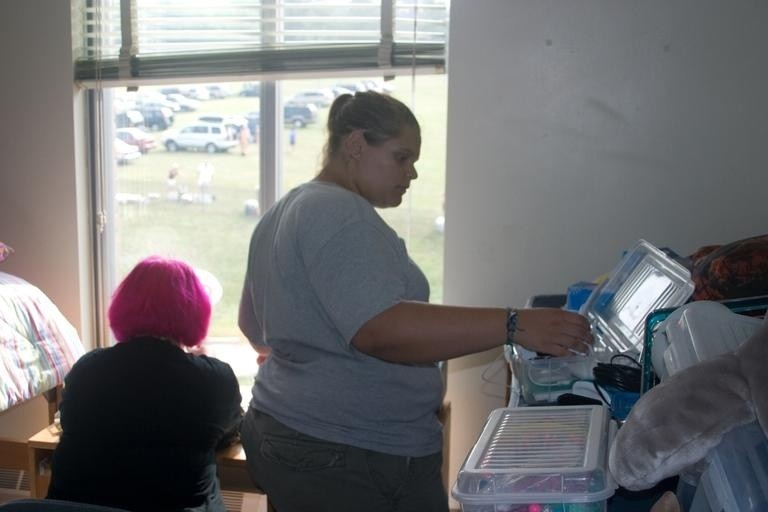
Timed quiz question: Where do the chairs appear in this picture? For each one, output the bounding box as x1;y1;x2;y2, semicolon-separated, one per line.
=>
0;498;126;512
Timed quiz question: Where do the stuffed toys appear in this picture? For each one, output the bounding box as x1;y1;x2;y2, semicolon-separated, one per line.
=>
607;308;768;492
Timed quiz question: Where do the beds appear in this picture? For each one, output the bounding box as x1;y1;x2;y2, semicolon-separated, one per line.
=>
0;266;85;471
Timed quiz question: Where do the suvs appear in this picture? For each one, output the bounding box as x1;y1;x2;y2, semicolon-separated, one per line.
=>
161;114;248;153
246;101;318;128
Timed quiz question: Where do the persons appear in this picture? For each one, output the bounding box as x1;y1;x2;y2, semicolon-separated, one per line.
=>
43;254;245;511
238;120;250;157
237;89;598;511
197;157;214;193
289;125;297;148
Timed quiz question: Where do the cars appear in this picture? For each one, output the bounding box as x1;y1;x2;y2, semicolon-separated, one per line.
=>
240;84;261;97
113;94;173;162
285;78;394;107
133;83;228;112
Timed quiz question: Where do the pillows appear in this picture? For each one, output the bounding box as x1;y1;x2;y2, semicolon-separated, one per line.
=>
0;241;15;263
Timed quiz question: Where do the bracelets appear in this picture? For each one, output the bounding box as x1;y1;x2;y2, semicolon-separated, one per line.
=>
505;307;519;346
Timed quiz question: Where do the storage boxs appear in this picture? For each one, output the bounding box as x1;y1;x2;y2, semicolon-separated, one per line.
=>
451;400;621;498
505;240;699;406
641;301;765;498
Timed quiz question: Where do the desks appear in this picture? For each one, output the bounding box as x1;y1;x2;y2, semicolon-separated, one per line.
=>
29;423;266;500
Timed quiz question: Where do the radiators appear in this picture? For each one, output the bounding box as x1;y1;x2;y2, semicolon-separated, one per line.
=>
0;469;267;512
